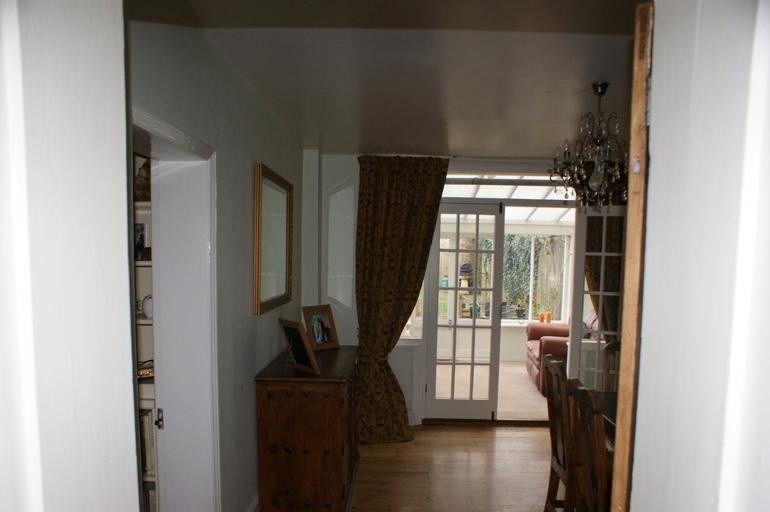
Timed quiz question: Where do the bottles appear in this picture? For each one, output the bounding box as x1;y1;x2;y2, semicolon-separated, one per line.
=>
442;275;448;288
539;311;552;323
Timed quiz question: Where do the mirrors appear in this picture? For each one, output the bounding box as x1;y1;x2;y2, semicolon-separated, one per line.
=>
254;158;295;314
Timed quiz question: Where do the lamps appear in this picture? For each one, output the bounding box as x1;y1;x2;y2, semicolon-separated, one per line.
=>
545;82;628;213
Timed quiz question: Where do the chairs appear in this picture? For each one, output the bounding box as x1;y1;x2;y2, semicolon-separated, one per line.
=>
525;308;604;399
539;367;621;511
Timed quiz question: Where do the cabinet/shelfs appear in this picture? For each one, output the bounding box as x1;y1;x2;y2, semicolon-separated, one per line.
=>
255;345;364;511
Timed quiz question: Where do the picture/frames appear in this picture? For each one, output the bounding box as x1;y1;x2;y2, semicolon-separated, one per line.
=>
278;303;339;377
